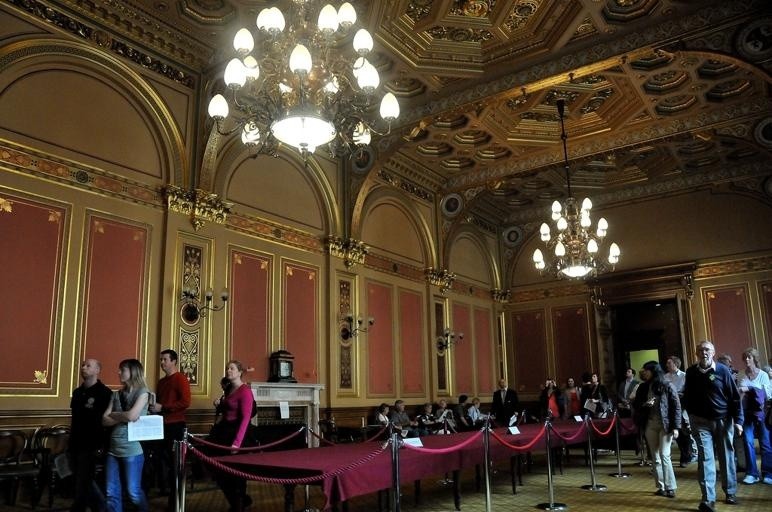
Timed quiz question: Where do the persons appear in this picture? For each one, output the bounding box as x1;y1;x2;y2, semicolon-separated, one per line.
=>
490;378;519;427
62;348;261;512
372;395;494;441
617;339;772;511
538;373;609;451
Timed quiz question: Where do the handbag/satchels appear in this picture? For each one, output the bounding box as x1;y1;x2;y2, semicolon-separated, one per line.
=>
238;400;258;420
141;440;155;465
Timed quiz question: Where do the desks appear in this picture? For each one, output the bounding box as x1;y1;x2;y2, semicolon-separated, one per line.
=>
206;415;641;511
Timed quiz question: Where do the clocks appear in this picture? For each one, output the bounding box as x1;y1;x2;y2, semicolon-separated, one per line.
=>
267;350;297;383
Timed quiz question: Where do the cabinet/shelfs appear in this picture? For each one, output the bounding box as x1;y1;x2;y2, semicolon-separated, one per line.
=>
245;381;326;449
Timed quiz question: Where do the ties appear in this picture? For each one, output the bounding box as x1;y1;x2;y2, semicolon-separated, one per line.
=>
503;391;506;402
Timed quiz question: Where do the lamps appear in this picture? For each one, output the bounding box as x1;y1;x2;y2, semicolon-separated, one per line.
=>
434;328;464;351
207;0;400;168
532;99;621;279
340;312;375;340
182;284;229;320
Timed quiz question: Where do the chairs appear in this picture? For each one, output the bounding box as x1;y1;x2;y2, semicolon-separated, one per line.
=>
34;424;73;508
0;430;39;507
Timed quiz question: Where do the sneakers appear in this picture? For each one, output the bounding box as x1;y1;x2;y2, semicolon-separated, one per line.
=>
655;487;666;498
763;476;772;485
741;473;761;485
666;489;678;497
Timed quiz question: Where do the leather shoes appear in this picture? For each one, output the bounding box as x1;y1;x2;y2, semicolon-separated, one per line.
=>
724;492;739;505
698;500;716;512
